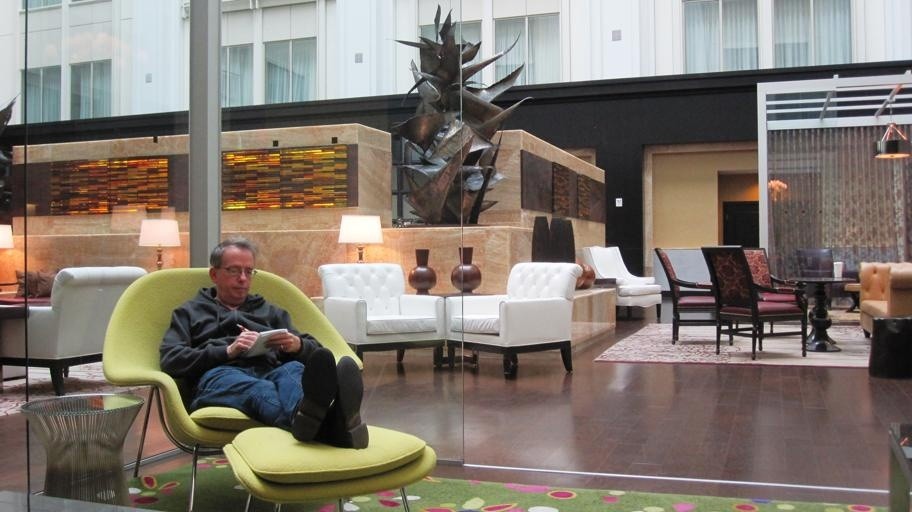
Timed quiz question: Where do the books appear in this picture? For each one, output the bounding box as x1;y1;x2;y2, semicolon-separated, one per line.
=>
240;328;287;358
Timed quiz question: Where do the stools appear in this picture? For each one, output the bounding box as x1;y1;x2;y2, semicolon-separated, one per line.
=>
223;428;437;512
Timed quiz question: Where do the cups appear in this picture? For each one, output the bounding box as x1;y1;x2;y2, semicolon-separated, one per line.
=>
832;261;843;279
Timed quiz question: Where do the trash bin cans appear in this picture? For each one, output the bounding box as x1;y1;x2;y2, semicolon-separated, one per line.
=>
869;317;912;377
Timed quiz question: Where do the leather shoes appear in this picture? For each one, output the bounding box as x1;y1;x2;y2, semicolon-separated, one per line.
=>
291;348;368;449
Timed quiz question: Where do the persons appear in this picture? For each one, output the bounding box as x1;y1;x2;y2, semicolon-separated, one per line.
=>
160;239;371;449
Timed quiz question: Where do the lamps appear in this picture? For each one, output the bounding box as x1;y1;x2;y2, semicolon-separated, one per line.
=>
339;214;383;262
137;219;183;268
872;103;911;160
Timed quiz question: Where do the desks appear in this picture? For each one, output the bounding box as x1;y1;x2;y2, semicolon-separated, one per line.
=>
785;275;859;353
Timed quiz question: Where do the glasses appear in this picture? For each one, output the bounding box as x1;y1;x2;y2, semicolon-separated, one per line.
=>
225;266;258;276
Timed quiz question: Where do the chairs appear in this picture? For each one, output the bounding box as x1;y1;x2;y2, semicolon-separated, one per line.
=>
445;259;584;380
582;244;663;320
860;262;911;338
308;261;446;369
655;247;809;358
0;264;148;397
103;267;365;511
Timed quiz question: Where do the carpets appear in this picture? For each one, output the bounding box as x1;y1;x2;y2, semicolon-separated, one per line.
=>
592;320;869;369
122;452;896;512
